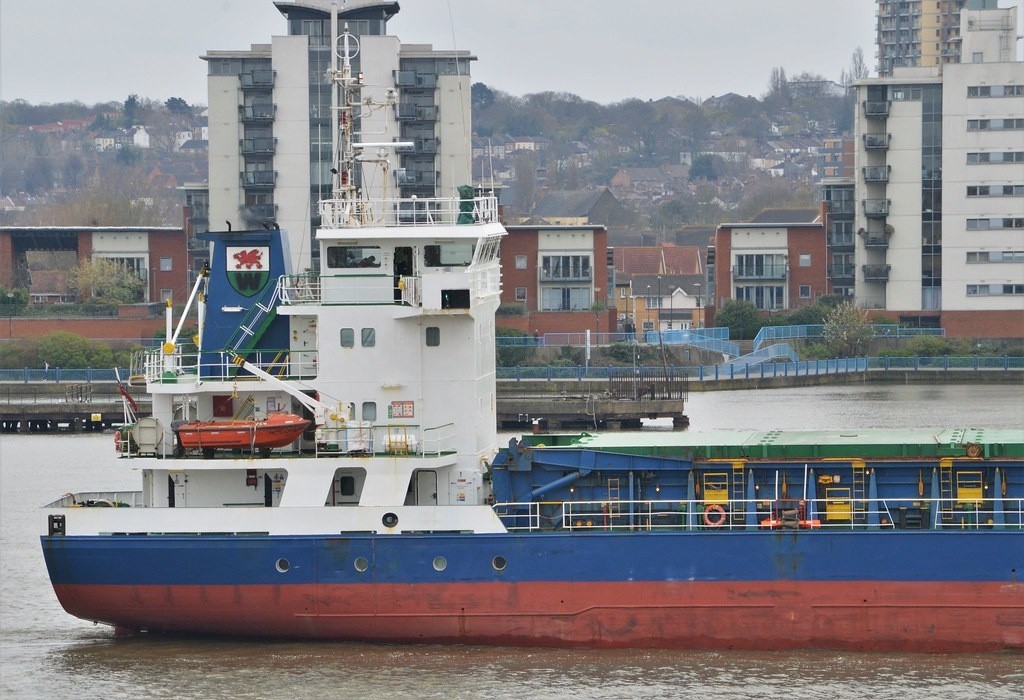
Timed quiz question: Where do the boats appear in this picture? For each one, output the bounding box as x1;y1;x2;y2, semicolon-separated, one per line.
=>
170;412;311;459
34;18;1024;651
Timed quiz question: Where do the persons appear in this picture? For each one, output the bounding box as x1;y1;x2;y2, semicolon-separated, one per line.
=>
348;249;379;268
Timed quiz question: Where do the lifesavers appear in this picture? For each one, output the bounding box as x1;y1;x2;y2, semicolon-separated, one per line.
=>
703;505;727;528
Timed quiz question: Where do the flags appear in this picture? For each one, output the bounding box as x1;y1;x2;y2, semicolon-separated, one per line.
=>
118;381;138;412
